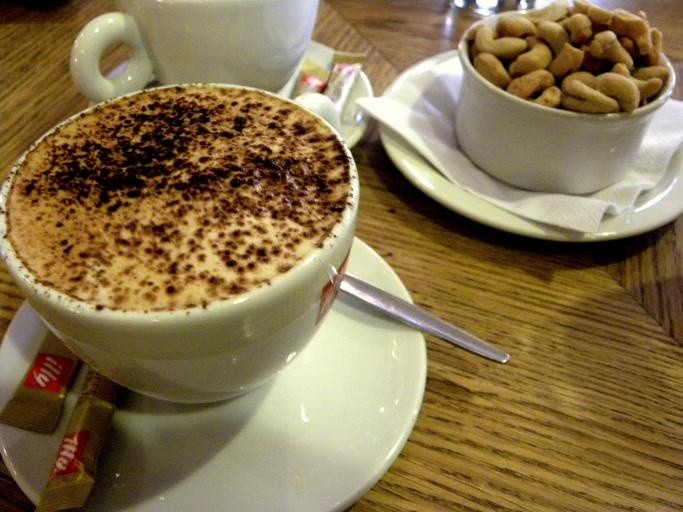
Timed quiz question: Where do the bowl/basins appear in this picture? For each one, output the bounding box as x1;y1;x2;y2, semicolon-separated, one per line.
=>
3;83;363;410
457;6;674;197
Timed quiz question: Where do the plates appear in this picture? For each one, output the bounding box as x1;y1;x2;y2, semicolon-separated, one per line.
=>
83;34;378;164
378;49;682;246
3;233;427;510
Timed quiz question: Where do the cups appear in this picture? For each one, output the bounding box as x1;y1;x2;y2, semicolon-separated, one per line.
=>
70;0;324;106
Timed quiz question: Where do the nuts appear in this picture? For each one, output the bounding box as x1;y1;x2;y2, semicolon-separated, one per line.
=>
470;0;670;115
